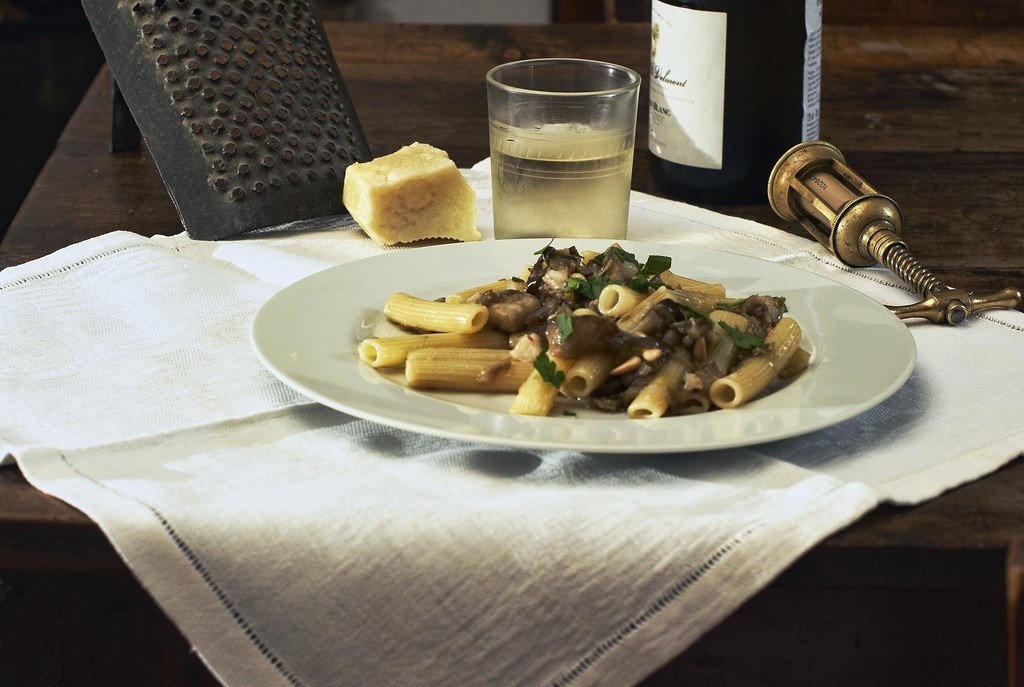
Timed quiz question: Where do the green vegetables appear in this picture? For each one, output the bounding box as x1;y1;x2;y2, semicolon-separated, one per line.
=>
531;234;790;419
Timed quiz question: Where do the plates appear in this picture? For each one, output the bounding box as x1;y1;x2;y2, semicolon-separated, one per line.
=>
249;238;917;453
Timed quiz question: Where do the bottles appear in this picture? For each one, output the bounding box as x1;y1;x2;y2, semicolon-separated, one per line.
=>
646;0;823;208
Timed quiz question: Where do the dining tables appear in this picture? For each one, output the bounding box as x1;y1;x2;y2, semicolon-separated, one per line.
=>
0;20;1024;687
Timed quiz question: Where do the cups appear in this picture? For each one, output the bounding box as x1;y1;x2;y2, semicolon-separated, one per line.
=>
485;57;642;241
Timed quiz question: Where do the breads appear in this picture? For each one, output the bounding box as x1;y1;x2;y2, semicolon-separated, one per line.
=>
341;140;481;247
